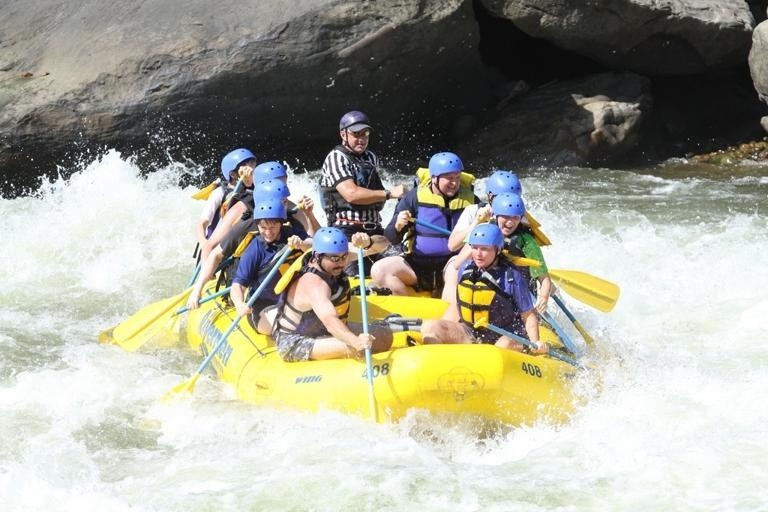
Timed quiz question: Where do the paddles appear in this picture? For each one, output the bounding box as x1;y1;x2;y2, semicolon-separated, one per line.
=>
409;219;619;314
162;247;292;399
96;287;229;346
112;201;303;353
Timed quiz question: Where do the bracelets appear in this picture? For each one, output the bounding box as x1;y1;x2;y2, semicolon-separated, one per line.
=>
384;189;391;200
241;182;255;190
363;238;373;250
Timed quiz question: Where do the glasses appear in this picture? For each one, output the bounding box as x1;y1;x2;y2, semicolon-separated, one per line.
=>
325;253;347;261
350;131;370;137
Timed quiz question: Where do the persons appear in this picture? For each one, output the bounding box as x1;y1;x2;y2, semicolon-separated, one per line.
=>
370;151;481;294
441;191;557;314
201;160;309;281
186;177;322;309
420;222;549;354
230;197;313;337
270;226;390;361
196;147;259;245
318;110;408;275
441;170;557;298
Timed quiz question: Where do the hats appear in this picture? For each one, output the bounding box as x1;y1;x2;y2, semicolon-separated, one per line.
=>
349;123;372;131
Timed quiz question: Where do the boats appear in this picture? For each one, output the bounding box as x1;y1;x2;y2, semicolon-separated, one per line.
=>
184;258;592;441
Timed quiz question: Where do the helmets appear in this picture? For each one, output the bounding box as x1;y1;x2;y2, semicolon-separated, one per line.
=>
487;170;522;196
254;198;287;220
468;224;504;247
429;152;464;176
253;161;287;186
221;148;257;180
254;178;290;203
340;111;373;129
312;228;348;254
491;192;525;216
